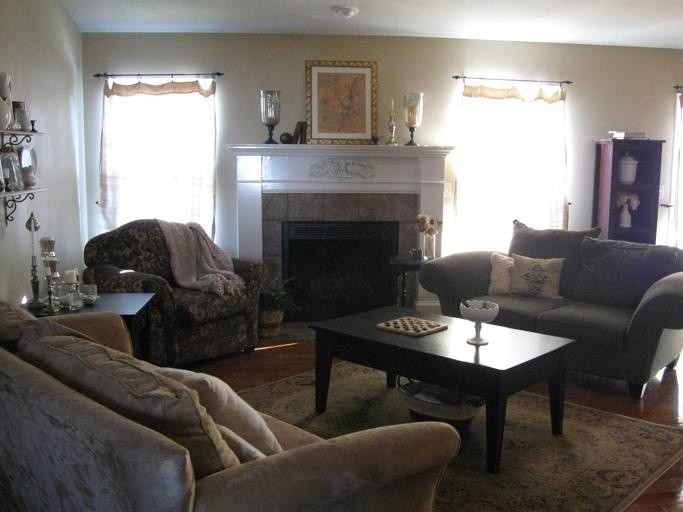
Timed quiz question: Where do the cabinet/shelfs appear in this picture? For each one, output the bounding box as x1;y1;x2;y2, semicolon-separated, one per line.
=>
591;139;666;244
1;130;46;227
28;294;155;359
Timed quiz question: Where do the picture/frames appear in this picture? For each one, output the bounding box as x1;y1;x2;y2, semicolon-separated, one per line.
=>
305;58;378;146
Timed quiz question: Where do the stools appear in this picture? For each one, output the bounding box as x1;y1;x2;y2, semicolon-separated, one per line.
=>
385;253;439;307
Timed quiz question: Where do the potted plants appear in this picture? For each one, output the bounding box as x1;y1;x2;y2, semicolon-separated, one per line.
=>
258;278;302;338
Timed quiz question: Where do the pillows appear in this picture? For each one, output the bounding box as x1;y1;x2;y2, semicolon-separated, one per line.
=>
215;422;266;461
489;254;513;295
92;346;284;454
513;253;566;300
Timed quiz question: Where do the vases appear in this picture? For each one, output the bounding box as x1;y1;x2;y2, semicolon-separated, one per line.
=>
417;232;426;261
430;236;436;260
621;203;631;227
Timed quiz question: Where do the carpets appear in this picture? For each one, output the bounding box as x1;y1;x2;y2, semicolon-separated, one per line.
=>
225;359;683;512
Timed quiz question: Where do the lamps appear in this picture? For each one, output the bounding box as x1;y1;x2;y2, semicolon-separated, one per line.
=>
21;296;27;305
25;212;43;309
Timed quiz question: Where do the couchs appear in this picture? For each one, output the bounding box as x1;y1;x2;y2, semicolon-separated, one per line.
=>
415;219;683;401
83;219;263;367
0;299;460;512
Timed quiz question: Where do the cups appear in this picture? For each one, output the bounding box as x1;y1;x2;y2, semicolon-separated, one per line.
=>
83;285;98;305
410;248;422;257
58;285;72;308
69;293;83;311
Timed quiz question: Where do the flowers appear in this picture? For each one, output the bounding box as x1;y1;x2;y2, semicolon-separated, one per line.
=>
414;214;429;232
427;217;441;236
617;192;640;210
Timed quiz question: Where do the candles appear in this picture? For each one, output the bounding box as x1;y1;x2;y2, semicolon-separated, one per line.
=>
268;99;274;116
409;104;416;125
64;271;76;284
73;267;79;273
45;266;52;275
391;98;394;110
